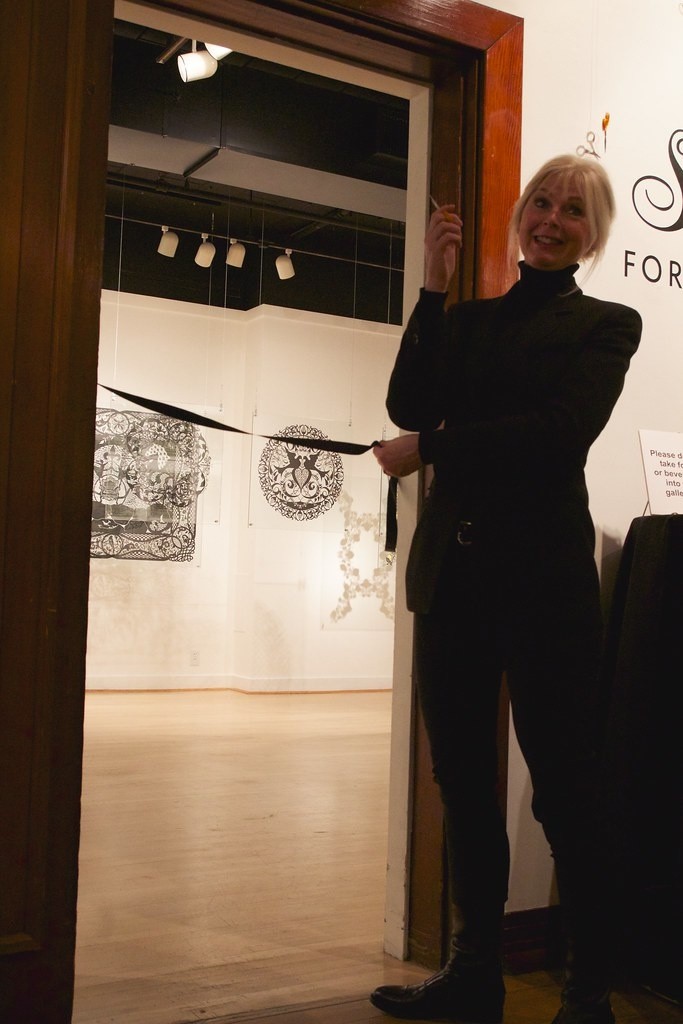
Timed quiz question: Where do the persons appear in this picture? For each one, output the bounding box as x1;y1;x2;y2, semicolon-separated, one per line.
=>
367;152;645;1023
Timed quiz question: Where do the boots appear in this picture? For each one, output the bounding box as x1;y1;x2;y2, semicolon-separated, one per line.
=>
549;933;615;1024
369;904;506;1024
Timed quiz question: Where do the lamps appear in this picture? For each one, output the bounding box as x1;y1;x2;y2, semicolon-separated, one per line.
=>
156;226;179;258
177;38;217;83
204;43;232;61
275;250;297;279
193;234;216;268
226;239;247;268
